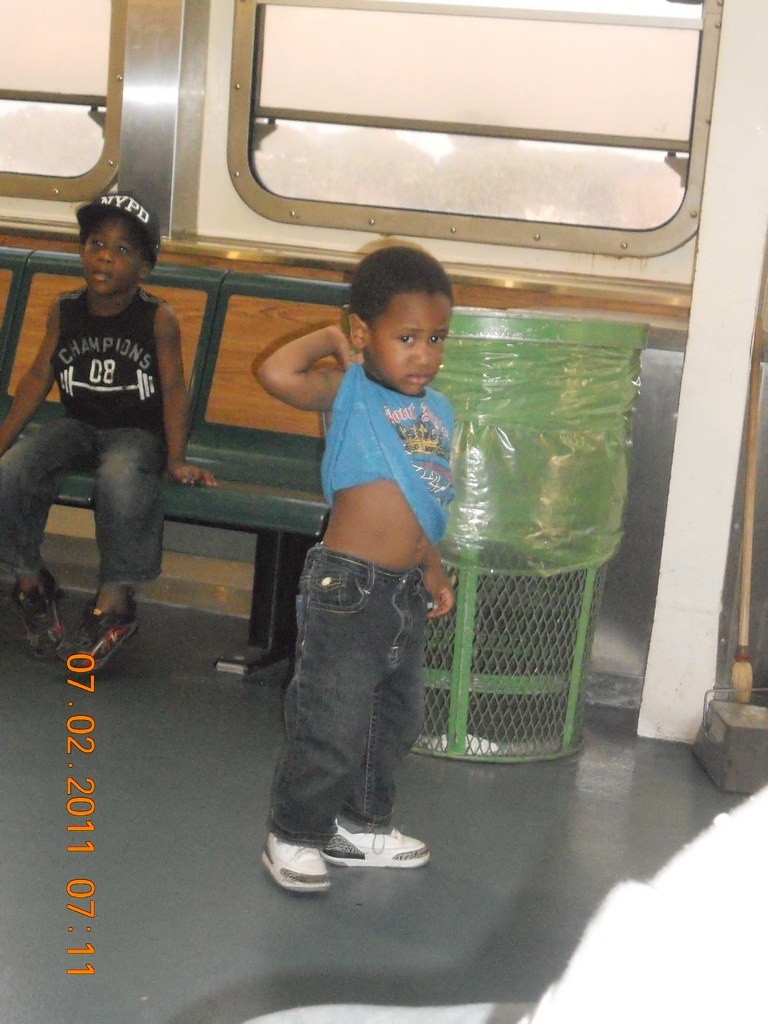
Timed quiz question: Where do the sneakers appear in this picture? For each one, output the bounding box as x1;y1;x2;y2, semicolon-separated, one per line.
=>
56;586;138;673
263;832;331;892
322;815;430;869
12;568;64;661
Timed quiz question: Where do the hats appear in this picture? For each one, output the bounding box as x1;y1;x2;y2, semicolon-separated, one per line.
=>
77;191;160;258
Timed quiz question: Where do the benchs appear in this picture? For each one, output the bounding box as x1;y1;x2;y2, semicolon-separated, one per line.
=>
0;246;351;675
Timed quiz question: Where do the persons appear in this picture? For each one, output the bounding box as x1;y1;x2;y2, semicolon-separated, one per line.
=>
254;248;456;895
0;192;217;673
